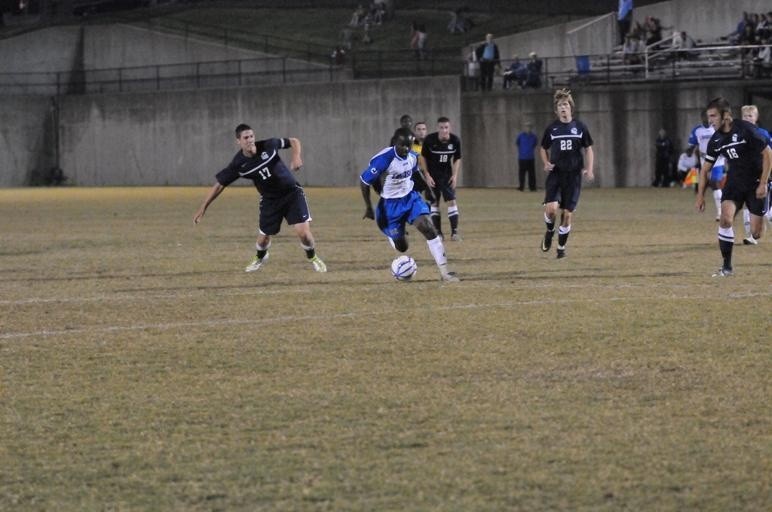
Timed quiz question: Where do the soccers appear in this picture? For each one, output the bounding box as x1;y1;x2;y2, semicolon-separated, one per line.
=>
391;256;417;280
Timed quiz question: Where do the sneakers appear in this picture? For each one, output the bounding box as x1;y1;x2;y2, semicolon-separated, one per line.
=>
308;254;327;272
441;272;458;282
557;248;566;258
744;234;757;244
246;251;269;271
712;266;734;277
451;234;461;241
542;230;555;252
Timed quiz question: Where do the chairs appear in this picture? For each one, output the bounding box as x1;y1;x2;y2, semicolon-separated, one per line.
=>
556;38;698;81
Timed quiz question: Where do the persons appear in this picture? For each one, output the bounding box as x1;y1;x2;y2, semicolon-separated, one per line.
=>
193;124;327;273
652;128;673;188
677;96;771;276
515;122;537;192
475;33;500;91
361;115;462;283
618;0;632;46
523;51;543;89
541;88;594;259
329;2;426;57
677;31;696;49
720;10;772;78
503;54;527;87
622;14;661;76
